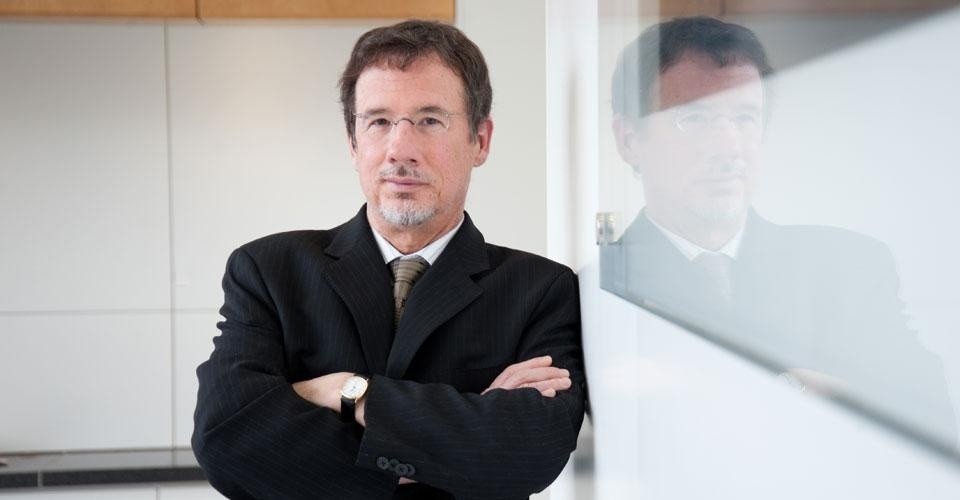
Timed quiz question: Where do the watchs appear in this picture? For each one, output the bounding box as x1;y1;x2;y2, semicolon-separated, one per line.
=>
340;372;371;422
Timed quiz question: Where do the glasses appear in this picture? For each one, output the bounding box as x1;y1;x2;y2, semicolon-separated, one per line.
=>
351;108;468;136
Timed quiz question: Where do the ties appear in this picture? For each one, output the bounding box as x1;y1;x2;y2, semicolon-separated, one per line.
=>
699;253;732;301
390;257;428;328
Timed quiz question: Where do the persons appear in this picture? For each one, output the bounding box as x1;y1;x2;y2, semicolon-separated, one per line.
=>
190;18;590;500
600;15;904;423
